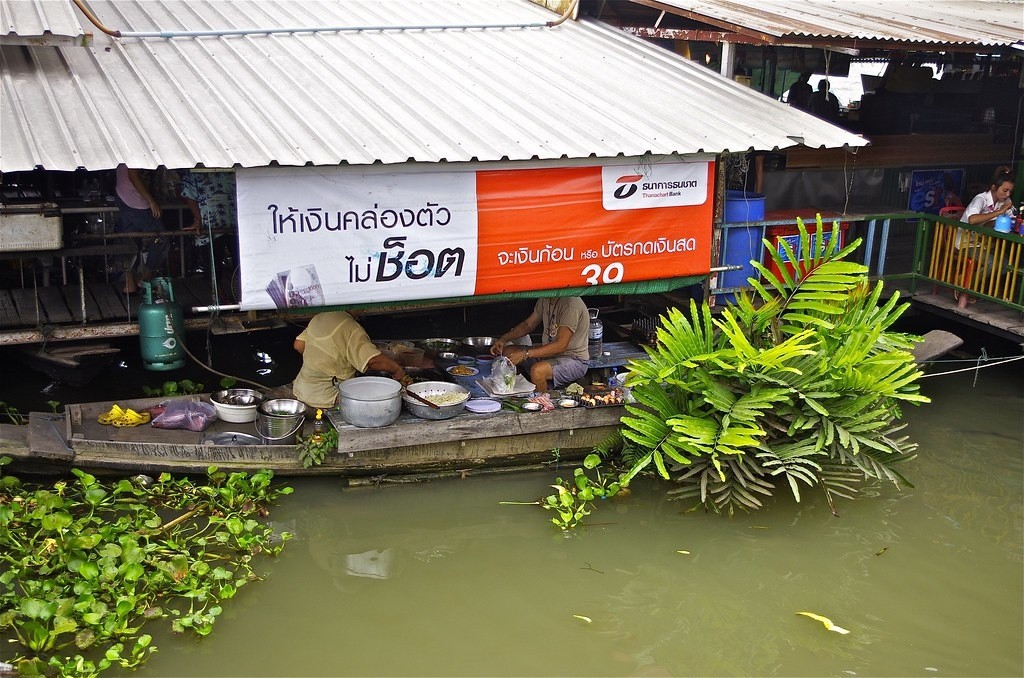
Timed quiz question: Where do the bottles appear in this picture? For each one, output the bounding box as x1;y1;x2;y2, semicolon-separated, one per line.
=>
607;368;618;386
313;409;324;441
1015;202;1024;233
587;308;603;360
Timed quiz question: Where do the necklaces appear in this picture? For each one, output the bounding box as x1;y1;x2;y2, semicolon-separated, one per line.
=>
546;298;560;335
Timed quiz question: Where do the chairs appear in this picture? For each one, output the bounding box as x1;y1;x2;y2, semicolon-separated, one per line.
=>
932;206;979;293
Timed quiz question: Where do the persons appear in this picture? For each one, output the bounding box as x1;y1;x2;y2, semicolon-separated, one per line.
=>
952;166;1015;300
788;71;813;110
489;297;590;394
285;308;405;408
113;162;202;294
179;169;241;306
809;80;840;116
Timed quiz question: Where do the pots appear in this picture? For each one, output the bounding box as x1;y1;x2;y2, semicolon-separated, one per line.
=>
331;375;404;428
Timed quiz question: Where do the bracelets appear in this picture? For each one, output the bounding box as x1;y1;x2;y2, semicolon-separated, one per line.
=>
523;346;530;360
391;368;403;373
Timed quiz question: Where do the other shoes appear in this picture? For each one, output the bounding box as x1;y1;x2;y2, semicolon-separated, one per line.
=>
958;295;968;309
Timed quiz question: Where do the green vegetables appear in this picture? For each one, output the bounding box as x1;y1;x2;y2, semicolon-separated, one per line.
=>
273;411;289;415
503;372;516;391
428;340;458;348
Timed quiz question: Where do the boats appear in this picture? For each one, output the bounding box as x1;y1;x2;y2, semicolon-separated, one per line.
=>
0;328;967;489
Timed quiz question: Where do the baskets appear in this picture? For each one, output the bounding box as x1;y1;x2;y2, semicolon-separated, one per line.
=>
399;348;425;366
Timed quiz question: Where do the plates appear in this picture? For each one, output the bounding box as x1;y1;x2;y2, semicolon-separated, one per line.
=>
521;402;542;410
465;400;501;413
558;399;579;408
446;365;479;376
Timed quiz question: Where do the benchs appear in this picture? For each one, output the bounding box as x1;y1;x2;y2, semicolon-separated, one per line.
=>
0;242;139;288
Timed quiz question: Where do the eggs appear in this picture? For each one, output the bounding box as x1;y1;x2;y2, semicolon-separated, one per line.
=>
581;394;622;406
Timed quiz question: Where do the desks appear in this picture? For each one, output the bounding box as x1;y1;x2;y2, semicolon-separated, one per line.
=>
43;201;194;277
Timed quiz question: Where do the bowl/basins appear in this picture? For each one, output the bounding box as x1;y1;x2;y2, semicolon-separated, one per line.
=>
209;388;269;424
373;341;424;373
476;355;495;364
463;336;498;348
456;356;475;365
420;337;461;352
439;353;458;361
262;399;306;416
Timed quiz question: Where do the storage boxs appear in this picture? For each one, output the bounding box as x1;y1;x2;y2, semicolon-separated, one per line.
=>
760;209;850;285
0;212;64;253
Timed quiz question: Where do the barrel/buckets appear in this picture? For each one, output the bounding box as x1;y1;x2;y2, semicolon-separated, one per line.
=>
676;189;767;306
254;398;308;446
